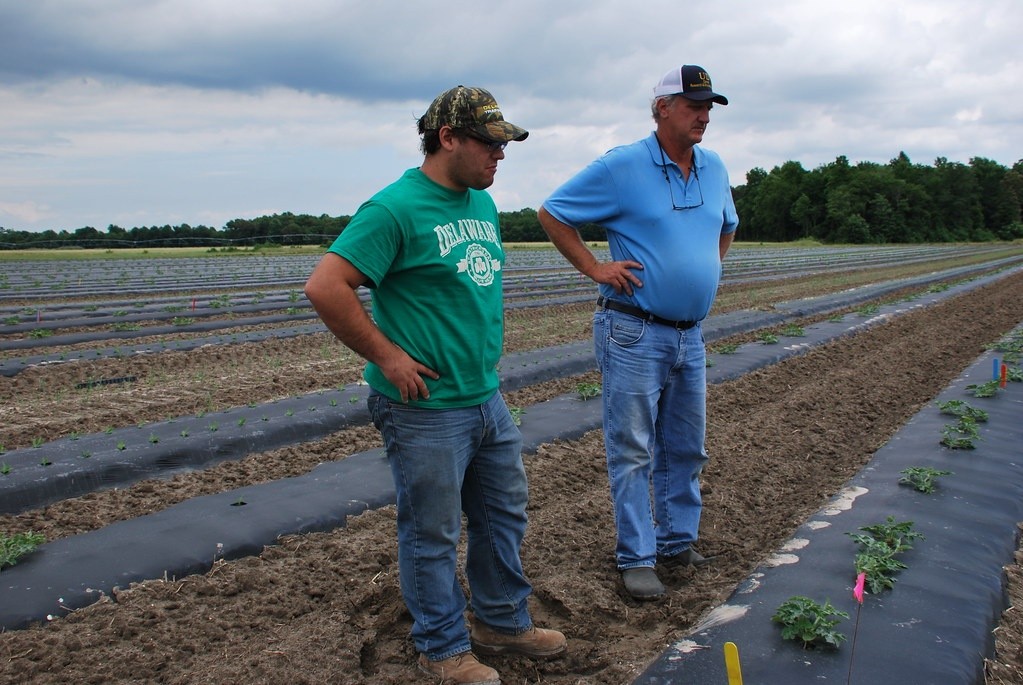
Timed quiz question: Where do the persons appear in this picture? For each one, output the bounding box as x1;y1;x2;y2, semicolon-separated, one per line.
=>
536;64;739;600
303;84;567;685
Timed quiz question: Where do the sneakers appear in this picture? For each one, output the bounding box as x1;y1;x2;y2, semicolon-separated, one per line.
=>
417;649;501;685
470;620;568;659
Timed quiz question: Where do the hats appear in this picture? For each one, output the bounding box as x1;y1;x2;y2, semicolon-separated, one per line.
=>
655;65;728;106
424;85;529;141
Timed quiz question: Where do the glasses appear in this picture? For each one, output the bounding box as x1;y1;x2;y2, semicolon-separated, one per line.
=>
465;133;508;153
663;167;704;210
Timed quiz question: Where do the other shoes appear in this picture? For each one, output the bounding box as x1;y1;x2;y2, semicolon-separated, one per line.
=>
674;548;706;567
622;567;665;600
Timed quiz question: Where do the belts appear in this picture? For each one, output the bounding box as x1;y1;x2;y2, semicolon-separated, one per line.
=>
597;297;697;329
